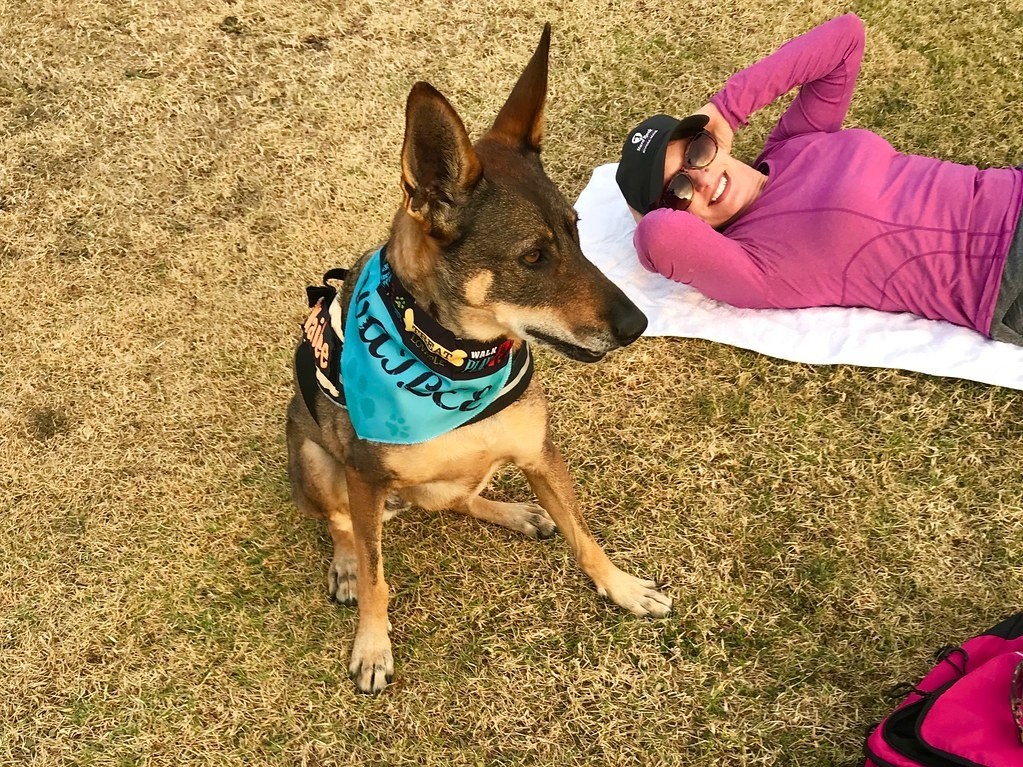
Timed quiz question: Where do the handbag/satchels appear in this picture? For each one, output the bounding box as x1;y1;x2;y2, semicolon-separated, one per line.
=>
865;614;1023;767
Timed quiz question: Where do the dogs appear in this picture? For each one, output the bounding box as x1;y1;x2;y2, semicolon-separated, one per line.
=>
285;23;673;698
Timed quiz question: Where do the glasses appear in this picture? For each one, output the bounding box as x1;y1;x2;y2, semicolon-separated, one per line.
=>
661;128;718;210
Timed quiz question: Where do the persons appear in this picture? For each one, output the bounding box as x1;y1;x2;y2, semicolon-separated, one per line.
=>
614;13;1023;347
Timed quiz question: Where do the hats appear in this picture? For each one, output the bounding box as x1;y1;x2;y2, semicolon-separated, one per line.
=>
616;114;710;214
